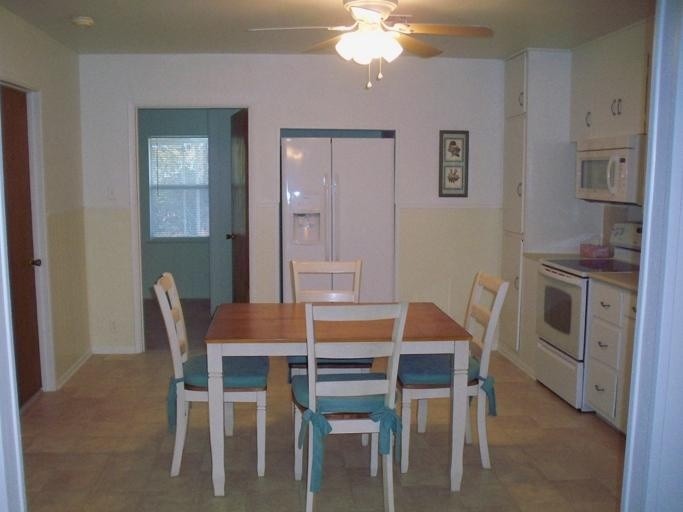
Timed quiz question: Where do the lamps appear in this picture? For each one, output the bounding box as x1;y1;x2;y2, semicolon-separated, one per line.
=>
333;21;404;89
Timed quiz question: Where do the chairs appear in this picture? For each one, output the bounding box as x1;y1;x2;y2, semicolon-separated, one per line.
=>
288;294;410;512
150;272;271;477
284;261;374;448
389;271;513;473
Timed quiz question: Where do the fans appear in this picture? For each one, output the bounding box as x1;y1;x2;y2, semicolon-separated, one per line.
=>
247;2;499;59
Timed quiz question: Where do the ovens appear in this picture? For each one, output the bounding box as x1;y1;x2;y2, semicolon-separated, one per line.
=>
532;267;585;411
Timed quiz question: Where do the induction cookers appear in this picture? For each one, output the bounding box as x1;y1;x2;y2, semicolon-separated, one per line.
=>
542;253;638;278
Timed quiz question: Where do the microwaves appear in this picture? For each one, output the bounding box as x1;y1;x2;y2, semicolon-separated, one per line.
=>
574;149;647;204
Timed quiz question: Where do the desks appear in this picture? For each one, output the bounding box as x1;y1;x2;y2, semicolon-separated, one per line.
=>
199;300;474;499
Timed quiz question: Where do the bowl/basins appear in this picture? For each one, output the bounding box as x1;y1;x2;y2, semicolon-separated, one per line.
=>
581;244;612;258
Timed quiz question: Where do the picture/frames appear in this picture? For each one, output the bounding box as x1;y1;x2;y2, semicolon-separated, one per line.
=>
439;129;468;199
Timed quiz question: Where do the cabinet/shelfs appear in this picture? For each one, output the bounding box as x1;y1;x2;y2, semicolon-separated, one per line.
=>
569;39;595;142
617;285;638;437
584;278;617;432
501;230;583;383
593;15;654;134
502;115;580;236
503;53;571;120
537;343;588;415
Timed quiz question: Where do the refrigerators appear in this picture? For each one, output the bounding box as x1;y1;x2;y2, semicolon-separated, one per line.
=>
280;136;396;303
